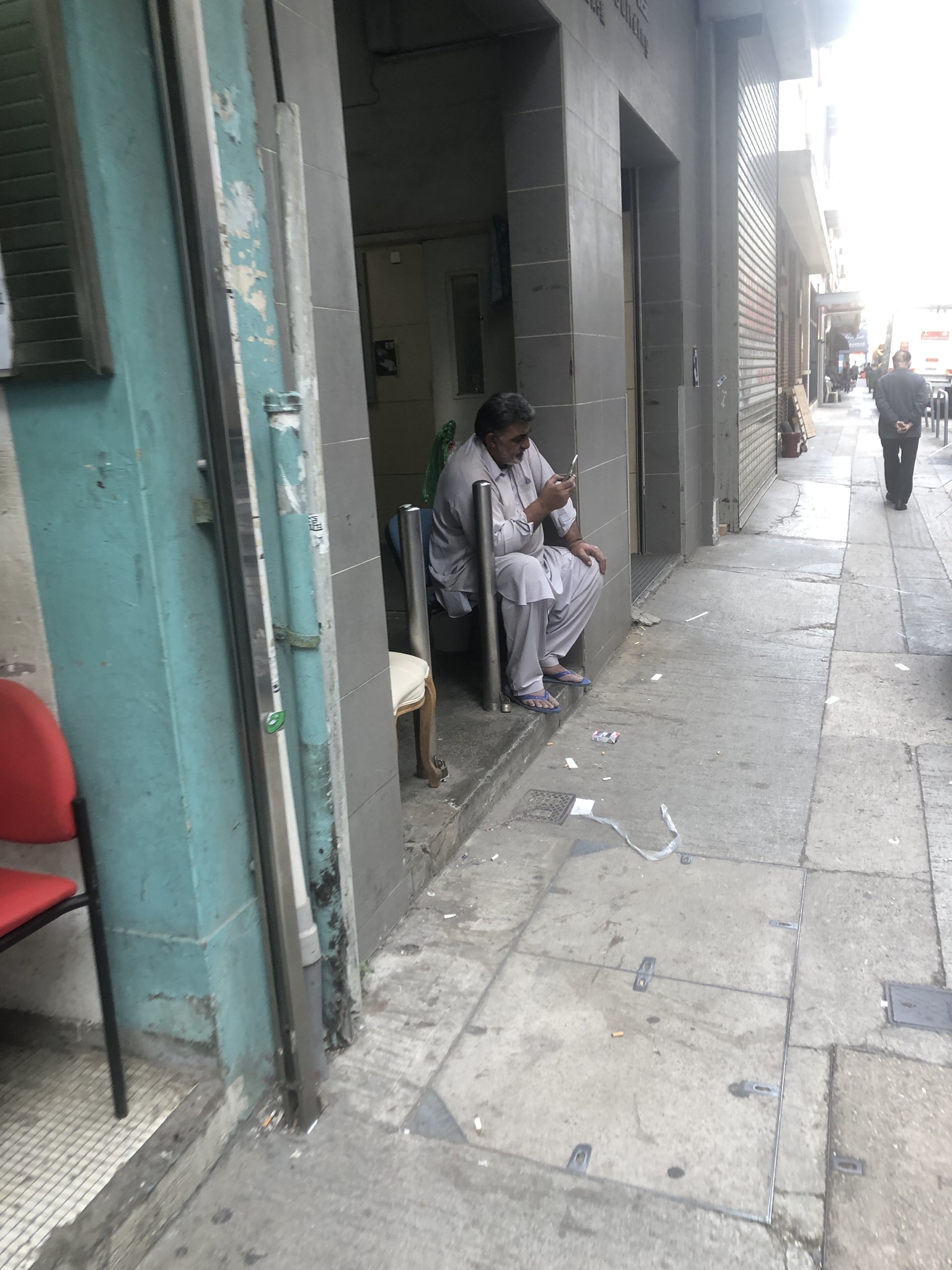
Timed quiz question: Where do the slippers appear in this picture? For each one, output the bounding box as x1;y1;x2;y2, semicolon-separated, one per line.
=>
542;669;591;687
505;685;561;713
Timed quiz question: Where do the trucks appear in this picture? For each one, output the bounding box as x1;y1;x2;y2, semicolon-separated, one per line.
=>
908;330;952;420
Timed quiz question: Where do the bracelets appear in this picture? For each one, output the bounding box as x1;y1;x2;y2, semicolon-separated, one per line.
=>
895;421;899;424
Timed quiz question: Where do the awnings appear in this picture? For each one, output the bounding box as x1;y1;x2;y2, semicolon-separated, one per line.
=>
834;334;850;350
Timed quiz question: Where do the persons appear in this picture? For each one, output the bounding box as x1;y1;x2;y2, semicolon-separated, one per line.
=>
874;350;929;510
864;363;873;387
860;363;864;375
429;392;606;714
843;363;852;393
851;365;859;382
825;360;838;392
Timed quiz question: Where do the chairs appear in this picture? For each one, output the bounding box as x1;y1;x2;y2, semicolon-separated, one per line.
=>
0;678;130;1121
383;508;512;688
824;380;838;402
388;651;444;790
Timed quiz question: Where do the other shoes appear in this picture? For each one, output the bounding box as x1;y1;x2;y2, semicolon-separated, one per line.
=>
886;492;897;503
895;499;907;510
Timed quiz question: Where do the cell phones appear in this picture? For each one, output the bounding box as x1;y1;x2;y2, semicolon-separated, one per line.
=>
562;454;578;482
